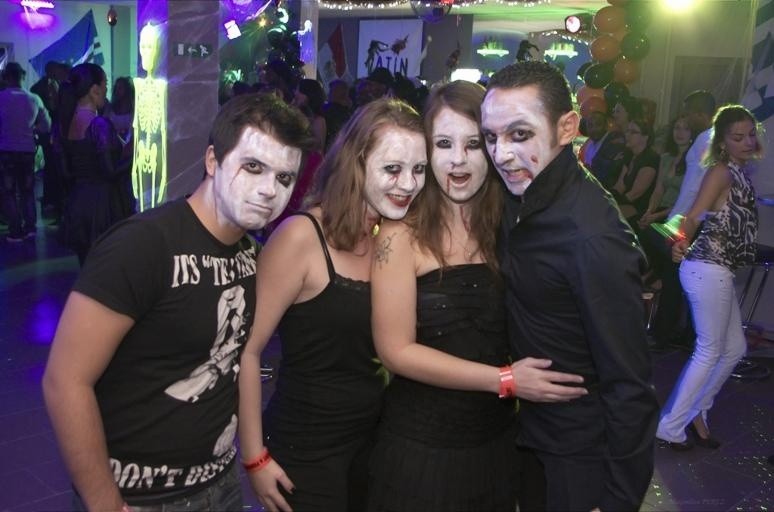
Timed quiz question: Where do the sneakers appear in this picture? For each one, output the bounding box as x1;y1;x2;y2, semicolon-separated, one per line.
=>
6;228;37;242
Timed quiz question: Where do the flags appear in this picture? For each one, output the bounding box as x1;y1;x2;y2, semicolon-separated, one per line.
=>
316;21;356;99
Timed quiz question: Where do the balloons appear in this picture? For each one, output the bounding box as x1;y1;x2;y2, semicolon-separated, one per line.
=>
588;0;654;64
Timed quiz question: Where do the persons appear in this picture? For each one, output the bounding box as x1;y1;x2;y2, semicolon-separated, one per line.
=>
0;49;766;512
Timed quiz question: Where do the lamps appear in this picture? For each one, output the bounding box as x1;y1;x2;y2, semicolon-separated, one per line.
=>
565;13;593;34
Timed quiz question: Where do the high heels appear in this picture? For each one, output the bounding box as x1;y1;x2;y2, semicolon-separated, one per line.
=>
656;421;720;450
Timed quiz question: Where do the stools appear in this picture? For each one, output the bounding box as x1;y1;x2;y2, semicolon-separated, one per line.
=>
728;244;773;381
